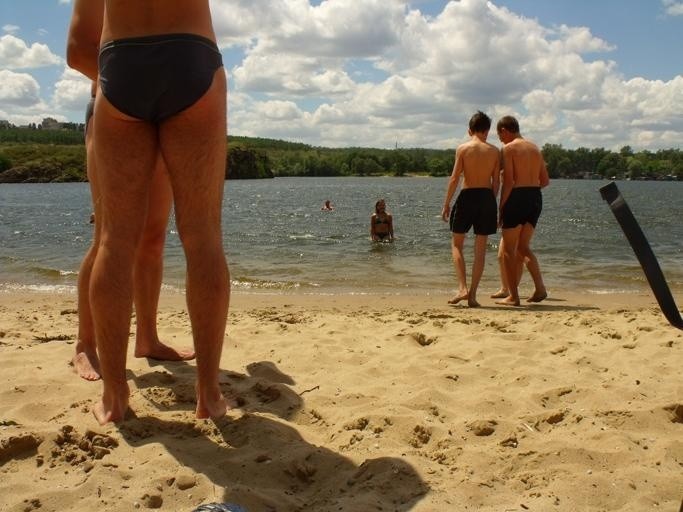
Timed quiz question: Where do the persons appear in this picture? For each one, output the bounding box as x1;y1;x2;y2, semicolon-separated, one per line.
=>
491;236;523;298
69;81;196;381
371;199;394;240
496;115;550;308
63;1;233;424
321;200;334;210
441;111;501;308
